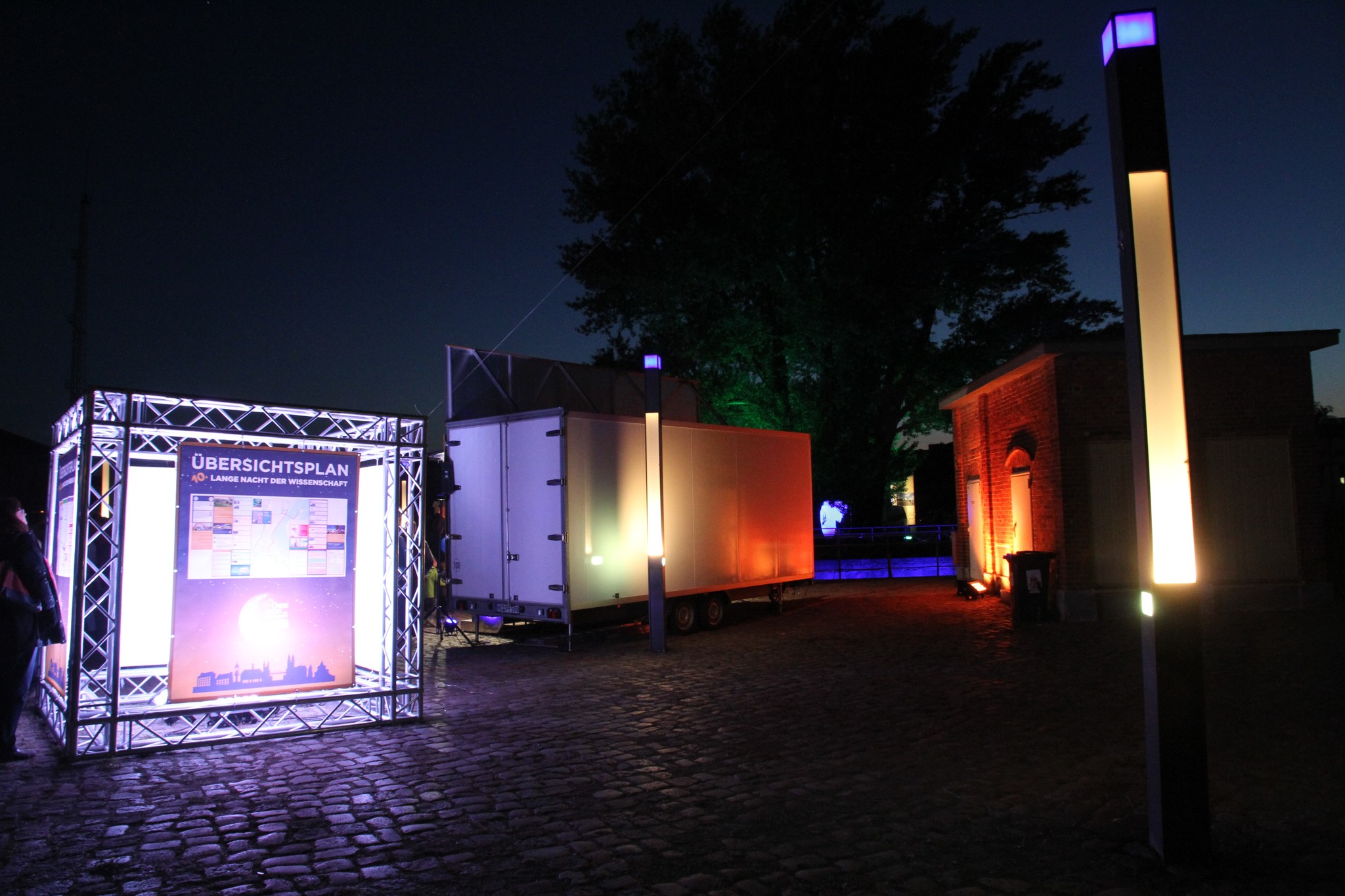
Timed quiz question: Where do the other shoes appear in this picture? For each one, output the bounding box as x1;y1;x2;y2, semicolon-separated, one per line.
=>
0;748;36;764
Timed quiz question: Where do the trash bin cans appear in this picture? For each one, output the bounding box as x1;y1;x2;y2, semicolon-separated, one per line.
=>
1002;550;1058;622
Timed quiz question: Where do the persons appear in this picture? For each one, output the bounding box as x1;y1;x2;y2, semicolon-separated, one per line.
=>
423;558;448;627
0;499;66;762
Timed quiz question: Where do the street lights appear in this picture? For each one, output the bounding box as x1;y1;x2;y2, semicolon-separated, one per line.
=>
1100;13;1214;867
643;351;669;652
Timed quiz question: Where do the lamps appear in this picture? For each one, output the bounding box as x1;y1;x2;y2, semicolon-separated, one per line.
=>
442;618;458;636
965;580;989;601
